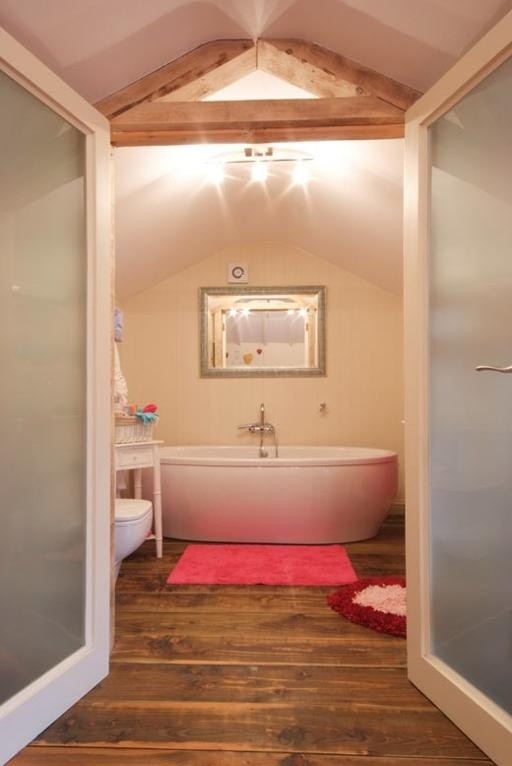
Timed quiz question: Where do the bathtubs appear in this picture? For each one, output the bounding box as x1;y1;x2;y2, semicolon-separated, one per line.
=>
132;446;398;545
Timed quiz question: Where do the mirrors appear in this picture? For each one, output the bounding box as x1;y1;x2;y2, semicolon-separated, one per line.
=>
198;285;326;379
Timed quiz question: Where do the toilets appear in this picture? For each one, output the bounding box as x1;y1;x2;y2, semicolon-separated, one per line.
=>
114;497;153;563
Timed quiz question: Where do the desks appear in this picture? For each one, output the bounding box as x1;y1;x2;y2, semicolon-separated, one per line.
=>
111;439;164;559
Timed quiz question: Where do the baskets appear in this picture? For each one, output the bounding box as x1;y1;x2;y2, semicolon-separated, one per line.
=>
115;415;159;444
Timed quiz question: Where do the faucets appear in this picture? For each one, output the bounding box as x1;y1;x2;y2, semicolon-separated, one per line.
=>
260;404;266;433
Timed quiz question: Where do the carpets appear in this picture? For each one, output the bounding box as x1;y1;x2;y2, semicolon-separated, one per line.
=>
165;542;407;640
